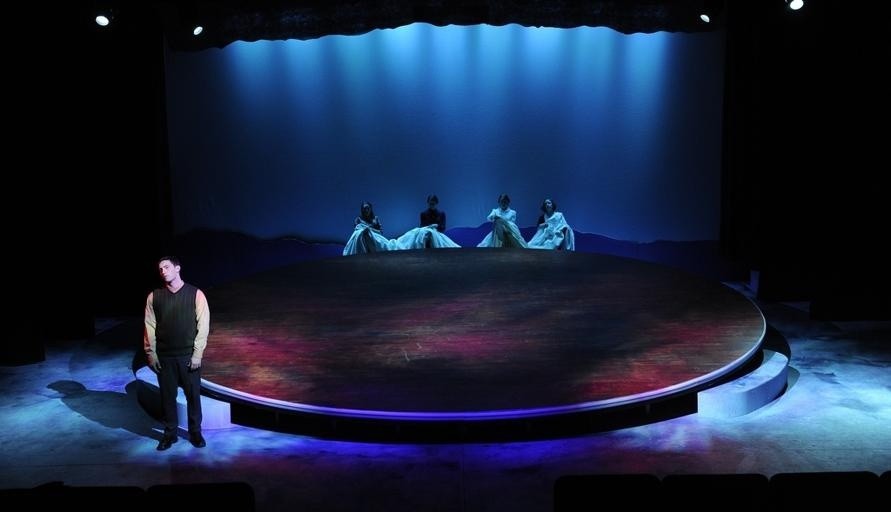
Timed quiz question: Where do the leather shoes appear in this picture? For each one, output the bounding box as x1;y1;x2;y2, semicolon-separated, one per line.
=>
157;435;178;450
191;433;207;448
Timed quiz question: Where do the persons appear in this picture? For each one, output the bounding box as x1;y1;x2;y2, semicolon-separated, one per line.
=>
538;198;568;251
420;194;446;247
144;257;212;452
489;193;518;246
354;201;385;253
